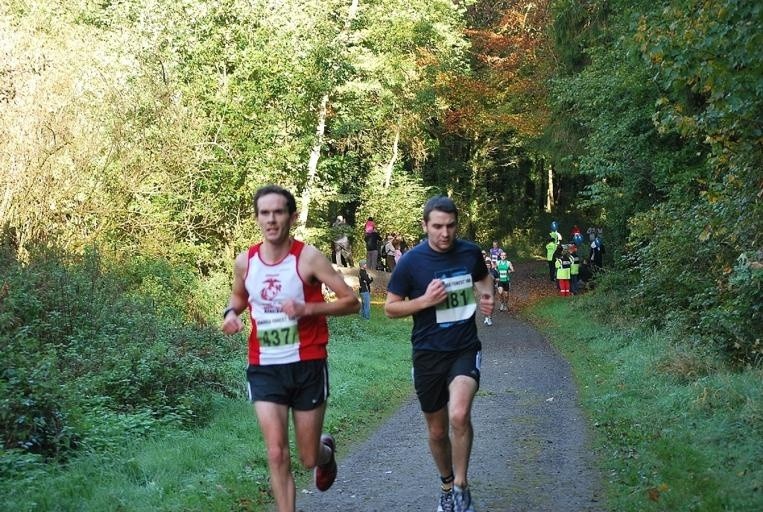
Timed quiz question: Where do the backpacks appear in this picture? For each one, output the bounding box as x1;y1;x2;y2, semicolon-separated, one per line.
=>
381;242;391;258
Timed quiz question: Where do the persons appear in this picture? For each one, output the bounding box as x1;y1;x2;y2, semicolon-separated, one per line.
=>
385;198;494;512
221;184;361;512
333;216;603;326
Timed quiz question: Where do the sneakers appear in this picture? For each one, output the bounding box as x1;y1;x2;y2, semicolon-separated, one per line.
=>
314;433;338;492
484;316;493;325
437;482;472;512
499;303;510;312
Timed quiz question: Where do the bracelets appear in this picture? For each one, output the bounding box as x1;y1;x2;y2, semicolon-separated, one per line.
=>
224;307;238;318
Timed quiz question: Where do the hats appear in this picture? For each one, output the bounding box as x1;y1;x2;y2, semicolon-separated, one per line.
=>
387;235;393;239
359;260;366;267
485;260;491;264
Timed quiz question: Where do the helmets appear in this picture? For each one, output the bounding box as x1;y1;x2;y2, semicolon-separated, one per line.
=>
550;221;558;232
567;243;578;255
595;238;603;248
560;244;568;256
572;232;585;246
